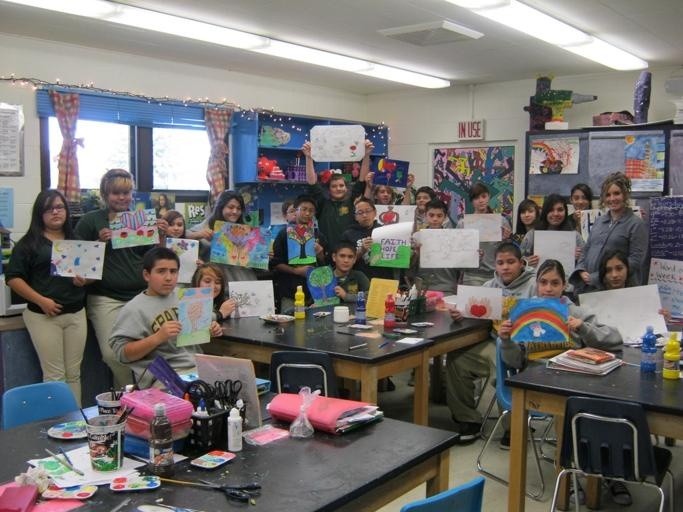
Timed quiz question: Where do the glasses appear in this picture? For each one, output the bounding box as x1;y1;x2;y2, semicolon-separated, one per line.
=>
43;206;65;212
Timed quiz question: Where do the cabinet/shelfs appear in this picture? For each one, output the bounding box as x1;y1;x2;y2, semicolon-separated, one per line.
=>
232;111;388;239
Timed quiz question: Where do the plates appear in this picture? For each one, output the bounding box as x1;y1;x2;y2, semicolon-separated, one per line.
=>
260;315;296;324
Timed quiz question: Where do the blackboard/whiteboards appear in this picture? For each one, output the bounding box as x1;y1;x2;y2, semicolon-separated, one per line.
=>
649;197;683;260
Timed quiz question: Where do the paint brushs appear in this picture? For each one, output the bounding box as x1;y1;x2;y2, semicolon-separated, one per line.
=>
115;404;134;425
110;385;124;401
129;368;148;393
80;408;89;424
44;445;84;476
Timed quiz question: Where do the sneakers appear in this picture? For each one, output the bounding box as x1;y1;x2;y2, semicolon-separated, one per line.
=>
499;430;510;450
569;475;585;504
460;422;481;441
609;481;632;504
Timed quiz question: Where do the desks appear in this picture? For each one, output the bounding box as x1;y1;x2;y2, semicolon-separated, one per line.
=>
504;325;683;512
283;302;492;400
213;314;435;427
1;391;460;512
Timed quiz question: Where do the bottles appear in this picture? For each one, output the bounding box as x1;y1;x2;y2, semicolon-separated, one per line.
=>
641;326;657;373
227;409;243;452
663;332;681;380
355;291;367;325
293;286;306;319
149;403;174;476
334;306;350;323
385;293;396;328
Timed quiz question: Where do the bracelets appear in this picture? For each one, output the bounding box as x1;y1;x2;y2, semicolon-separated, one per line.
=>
213;310;222;321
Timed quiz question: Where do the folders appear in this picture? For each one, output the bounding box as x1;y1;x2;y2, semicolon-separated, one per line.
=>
269;391;370;436
545;349;626;376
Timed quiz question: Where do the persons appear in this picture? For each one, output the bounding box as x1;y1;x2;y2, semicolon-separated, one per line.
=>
192;263;236;323
162;211;185;238
106;247;223;391
159;194;168;217
265;136;648;450
184;190;258;301
74;169;168;390
4;189;88;410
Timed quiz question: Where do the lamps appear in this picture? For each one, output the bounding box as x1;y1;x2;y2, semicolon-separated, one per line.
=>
0;0;450;89
445;0;648;71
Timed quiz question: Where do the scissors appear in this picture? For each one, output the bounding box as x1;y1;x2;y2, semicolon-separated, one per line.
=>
199;478;262;503
215;379;242;409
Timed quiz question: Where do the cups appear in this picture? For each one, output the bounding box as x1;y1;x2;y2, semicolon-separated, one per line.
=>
85;415;127;471
95;391;128;415
401;296;427;325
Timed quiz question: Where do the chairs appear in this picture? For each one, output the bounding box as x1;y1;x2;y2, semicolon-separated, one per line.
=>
400;475;486;512
270;351;340;398
473;376;557;442
1;380;79;430
476;335;557;501
551;396;674;512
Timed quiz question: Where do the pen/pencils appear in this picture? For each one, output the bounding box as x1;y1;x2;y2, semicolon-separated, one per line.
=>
348;343;368;350
336;331;353;335
379;342;388;348
